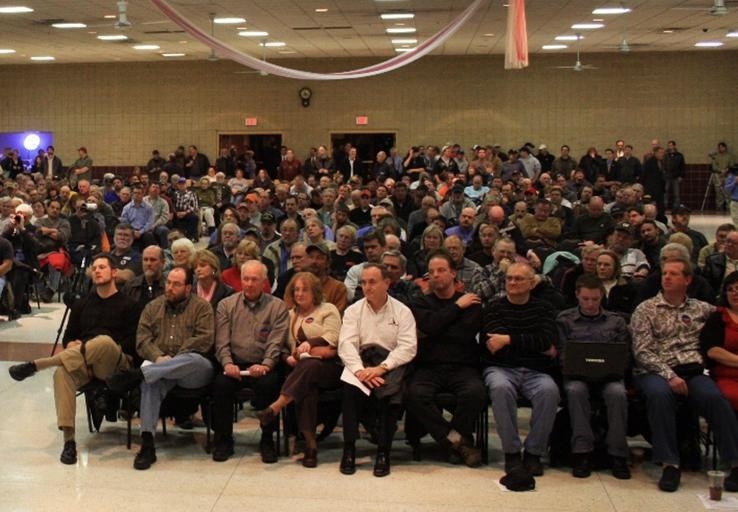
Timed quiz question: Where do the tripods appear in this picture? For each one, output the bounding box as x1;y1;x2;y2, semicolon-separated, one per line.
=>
700;160;732;214
49;258;92;359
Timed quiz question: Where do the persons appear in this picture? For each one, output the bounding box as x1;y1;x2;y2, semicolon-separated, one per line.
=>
2;133;737;492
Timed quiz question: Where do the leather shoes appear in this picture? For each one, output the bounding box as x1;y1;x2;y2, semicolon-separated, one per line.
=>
60;439;77;465
609;454;631;479
104;370;142;391
571;449;598;479
340;446;356;475
503;450;521;474
659;464;681;492
213;435;234;462
374;451;392;477
523;449;545;476
302;446;319;468
133;445;157;470
8;360;40;381
256;406;276;429
258;439;279;464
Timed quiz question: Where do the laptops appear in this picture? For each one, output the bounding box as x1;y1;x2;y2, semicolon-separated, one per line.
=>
564;340;627;383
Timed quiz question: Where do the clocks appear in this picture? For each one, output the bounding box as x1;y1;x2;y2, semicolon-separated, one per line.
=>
299;87;312;106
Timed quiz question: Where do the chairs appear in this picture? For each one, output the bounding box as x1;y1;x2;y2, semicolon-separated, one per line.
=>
0;246;72;321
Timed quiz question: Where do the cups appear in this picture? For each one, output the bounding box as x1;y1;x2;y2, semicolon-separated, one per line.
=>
707;471;725;501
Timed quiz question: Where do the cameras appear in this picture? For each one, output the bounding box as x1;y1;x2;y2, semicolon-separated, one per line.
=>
708;152;718;160
81;202;98;213
73;241;98;259
15;215;26;224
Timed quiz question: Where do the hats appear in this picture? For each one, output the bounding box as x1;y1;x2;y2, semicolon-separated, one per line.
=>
244;193;258;203
360;189;371;198
305;242;331;256
236;202;249;211
538;143;548;150
260;212;276;223
470;144;481;150
176;176;186;183
245;227;259;238
671;203;691;214
525;187;537;196
378;198;393;208
452;184;464;194
614;222;632;235
441;145;452;152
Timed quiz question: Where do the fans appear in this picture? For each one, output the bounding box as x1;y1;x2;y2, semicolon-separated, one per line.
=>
670;0;738;16
207;12;219;61
602;13;647;52
232;40;268;75
551;33;600;71
88;0;170;30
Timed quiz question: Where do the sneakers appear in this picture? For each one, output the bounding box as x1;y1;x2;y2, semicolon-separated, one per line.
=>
445;429;474;466
450;432;485;468
